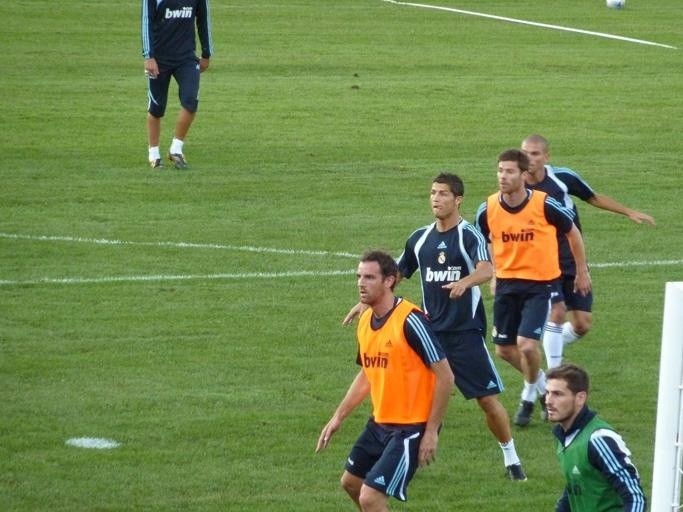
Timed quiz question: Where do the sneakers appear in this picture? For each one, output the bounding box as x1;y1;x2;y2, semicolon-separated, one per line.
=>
165;148;189;170
535;388;549;422
149;157;165;169
507;464;527;483
513;400;533;426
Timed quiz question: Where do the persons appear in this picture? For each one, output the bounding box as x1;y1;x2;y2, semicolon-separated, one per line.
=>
342;174;528;483
142;0;212;170
546;364;647;511
474;148;592;427
523;134;659;369
313;249;457;512
